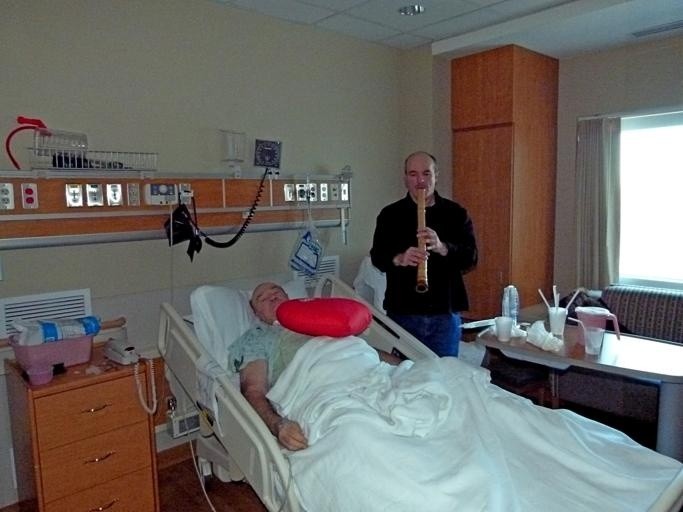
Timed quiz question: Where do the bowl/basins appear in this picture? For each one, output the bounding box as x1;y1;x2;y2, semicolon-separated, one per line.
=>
26;364;53;386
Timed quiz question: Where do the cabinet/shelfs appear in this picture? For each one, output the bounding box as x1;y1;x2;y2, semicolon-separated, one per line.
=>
2;342;161;512
450;44;553;321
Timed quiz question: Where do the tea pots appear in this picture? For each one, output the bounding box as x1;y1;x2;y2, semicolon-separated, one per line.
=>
574;306;620;347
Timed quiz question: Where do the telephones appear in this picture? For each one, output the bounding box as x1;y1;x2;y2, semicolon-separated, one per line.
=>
103;339;140;366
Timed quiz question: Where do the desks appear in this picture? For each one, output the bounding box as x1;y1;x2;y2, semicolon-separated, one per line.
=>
484;321;683;462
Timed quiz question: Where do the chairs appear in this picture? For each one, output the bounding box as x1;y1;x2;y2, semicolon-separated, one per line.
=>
545;285;683;421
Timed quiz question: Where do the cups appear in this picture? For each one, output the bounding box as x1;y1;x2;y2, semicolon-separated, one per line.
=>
548;306;566;335
494;316;513;342
584;327;603;356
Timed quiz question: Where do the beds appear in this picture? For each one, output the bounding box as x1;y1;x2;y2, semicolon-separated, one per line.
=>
148;275;682;511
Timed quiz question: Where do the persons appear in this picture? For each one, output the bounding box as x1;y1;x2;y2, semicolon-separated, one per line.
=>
368;147;479;358
225;281;406;455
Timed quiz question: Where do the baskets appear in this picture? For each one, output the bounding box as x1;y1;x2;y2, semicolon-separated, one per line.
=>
27;147;157;174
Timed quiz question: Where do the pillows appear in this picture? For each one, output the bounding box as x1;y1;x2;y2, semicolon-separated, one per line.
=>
276;293;374;340
188;280;324;369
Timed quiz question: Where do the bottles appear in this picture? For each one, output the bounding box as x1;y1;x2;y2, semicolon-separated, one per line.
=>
501;285;519;328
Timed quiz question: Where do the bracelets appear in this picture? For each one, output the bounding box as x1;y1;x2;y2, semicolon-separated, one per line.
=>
273;417;288;433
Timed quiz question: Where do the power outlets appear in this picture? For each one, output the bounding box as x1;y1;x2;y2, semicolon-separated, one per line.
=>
0;183;14;209
